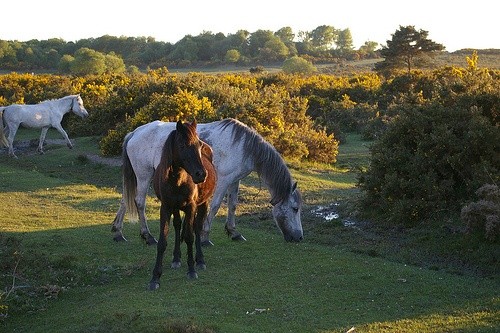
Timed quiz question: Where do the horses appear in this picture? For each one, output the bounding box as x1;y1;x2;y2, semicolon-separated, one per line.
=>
111;118;305;244
1;91;89;158
147;119;217;290
